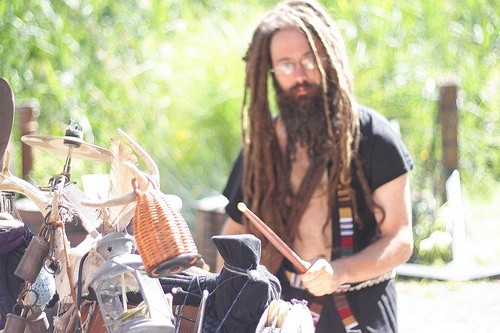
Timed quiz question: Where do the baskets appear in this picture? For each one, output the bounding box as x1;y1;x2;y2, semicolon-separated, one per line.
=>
131;173;199;277
27;266;55;307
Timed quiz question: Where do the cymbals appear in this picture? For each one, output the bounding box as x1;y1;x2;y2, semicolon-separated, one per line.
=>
1;173;50;204
19;134;113;162
0;78;14;160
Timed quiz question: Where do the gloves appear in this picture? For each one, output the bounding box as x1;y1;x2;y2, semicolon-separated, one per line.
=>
300;258;339;297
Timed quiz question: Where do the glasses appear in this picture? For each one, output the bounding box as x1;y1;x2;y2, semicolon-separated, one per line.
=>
270;56;328;75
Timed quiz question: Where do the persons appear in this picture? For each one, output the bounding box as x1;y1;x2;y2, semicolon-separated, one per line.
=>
210;1;414;333
